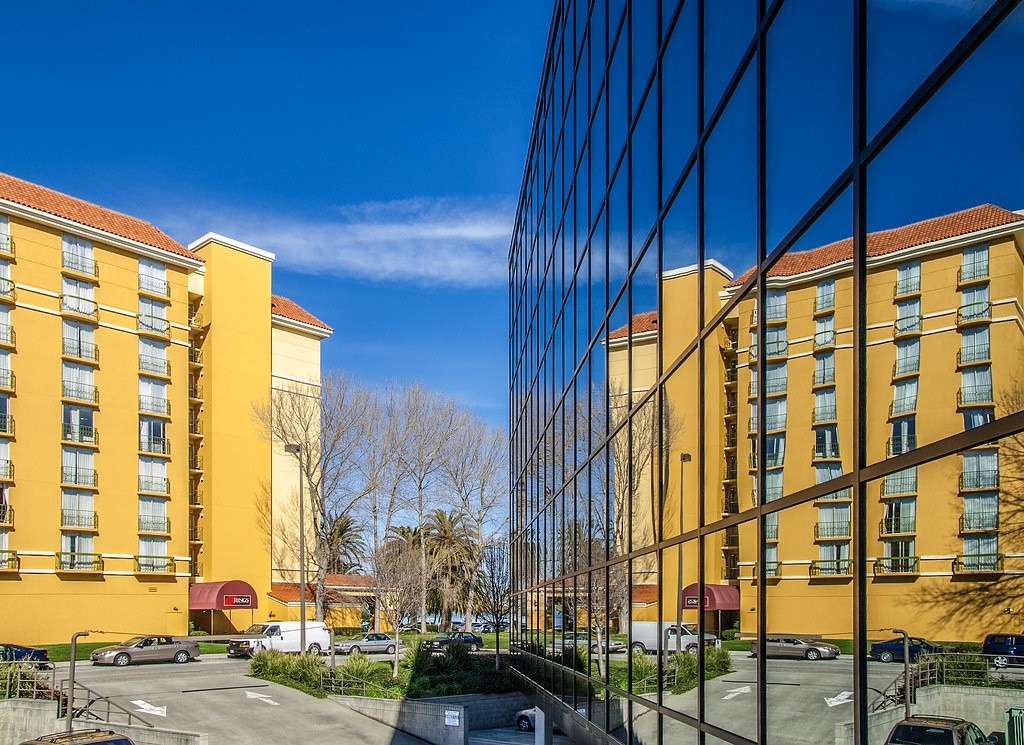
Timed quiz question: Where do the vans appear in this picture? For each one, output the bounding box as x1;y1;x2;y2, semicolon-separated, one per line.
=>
982;634;1024;666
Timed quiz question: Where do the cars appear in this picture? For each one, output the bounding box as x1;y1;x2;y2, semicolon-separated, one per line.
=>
90;635;202;664
0;643;49;670
552;632;626;655
870;637;960;663
514;706;559;732
334;632;402;654
420;631;484;653
750;633;840;662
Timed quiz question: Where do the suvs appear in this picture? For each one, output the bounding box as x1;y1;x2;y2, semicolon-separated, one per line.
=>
882;713;999;745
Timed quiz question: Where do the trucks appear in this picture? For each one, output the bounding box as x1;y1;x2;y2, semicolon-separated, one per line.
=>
227;621;331;658
631;620;717;656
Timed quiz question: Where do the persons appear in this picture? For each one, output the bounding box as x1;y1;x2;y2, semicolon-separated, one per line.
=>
371;635;376;641
145;639;153;646
